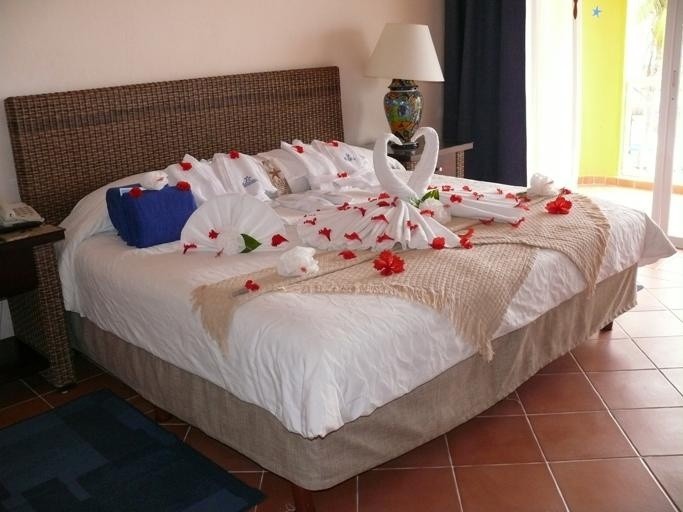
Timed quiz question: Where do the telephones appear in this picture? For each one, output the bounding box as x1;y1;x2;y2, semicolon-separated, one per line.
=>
0;200;45;228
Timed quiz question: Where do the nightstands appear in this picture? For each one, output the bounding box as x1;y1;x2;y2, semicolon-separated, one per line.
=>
362;132;473;181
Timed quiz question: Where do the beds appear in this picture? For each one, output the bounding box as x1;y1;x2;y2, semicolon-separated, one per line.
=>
5;62;677;508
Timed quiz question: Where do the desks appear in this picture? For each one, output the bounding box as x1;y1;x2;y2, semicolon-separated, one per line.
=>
0;221;85;393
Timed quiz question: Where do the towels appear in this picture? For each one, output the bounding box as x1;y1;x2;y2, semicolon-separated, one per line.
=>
296;122;527;250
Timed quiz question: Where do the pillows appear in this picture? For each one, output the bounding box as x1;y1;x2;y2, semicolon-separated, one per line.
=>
87;128;367;252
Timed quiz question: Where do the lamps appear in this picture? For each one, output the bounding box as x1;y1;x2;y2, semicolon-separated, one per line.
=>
358;23;445;151
